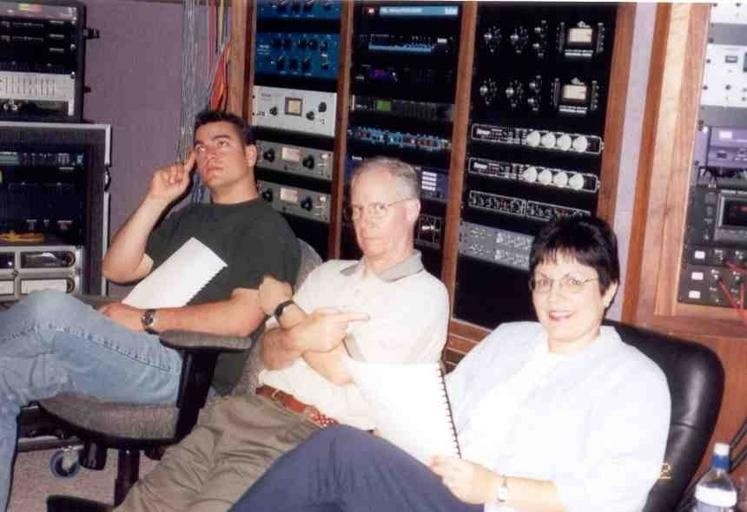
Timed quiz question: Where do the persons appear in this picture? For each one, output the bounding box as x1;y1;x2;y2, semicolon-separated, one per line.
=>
226;214;672;512
107;155;449;512
0;109;301;512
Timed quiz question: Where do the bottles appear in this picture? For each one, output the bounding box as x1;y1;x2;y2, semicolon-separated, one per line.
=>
694;441;739;512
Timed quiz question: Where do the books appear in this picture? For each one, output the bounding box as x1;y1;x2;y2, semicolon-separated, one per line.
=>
120;235;229;309
334;356;465;465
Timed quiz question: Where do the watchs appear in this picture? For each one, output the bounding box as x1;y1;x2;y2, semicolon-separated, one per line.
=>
141;308;159;335
276;300;294;320
498;473;509;503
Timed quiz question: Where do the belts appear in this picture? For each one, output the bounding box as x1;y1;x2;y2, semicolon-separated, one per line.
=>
255;384;339;431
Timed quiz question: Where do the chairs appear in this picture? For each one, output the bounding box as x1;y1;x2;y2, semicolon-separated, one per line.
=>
601;319;725;512
37;239;321;512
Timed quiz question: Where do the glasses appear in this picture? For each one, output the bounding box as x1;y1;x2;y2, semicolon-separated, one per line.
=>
343;197;415;221
527;272;600;295
